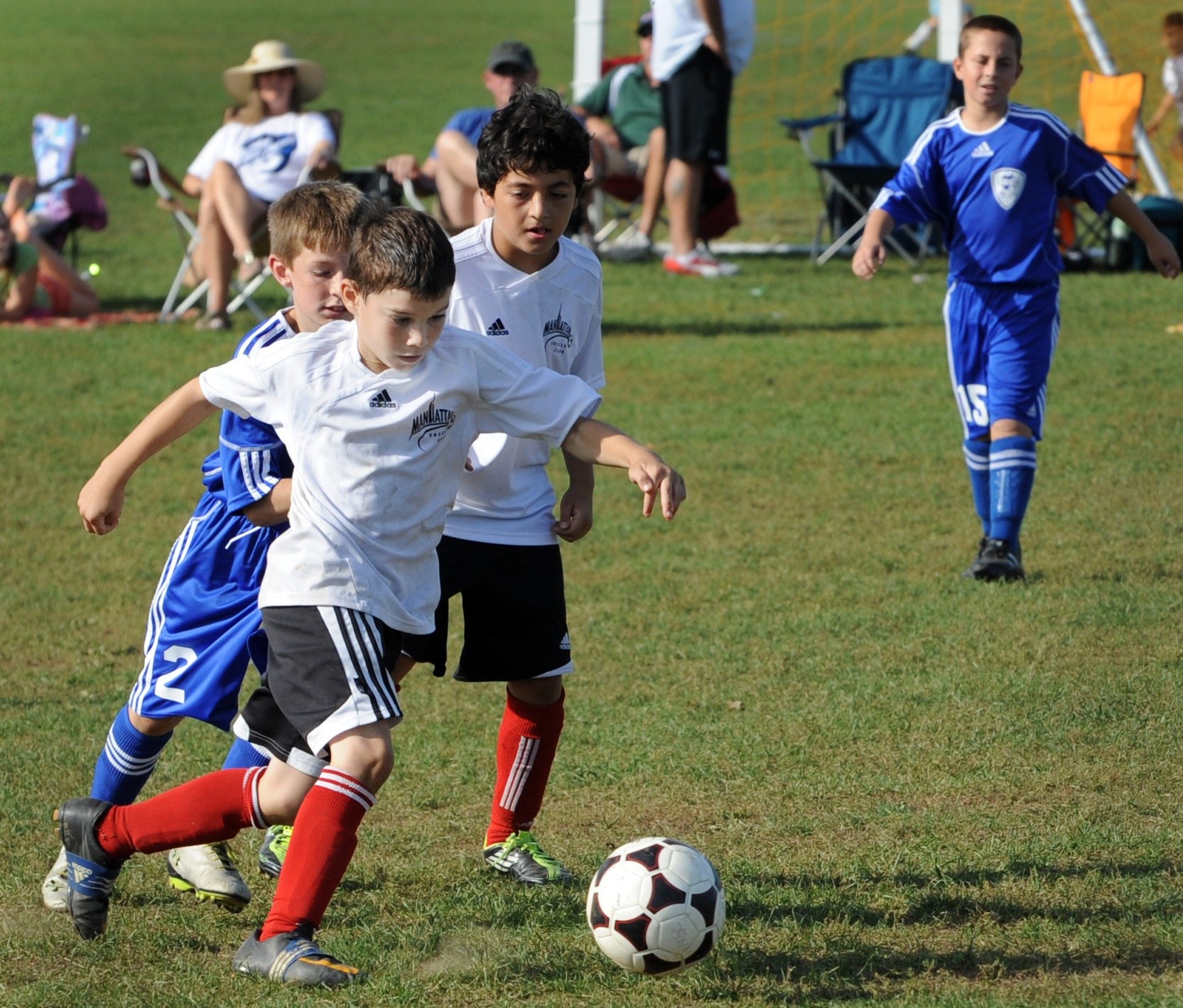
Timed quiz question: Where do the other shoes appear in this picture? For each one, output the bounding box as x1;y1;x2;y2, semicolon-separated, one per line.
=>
597;232;651;262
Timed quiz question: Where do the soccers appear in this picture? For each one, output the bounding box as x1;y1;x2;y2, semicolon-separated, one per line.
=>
585;834;728;979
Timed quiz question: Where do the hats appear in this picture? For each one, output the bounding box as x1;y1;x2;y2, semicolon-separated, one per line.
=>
490;41;534;74
223;40;327;105
636;11;652;34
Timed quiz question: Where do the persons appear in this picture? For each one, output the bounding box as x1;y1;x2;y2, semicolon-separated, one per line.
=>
387;0;756;276
41;178;381;914
256;79;607;885
52;208;685;986
0;40;337;329
1145;11;1183;165
850;15;1180;581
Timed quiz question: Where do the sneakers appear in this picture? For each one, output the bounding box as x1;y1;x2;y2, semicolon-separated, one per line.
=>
482;828;573;887
256;824;294;881
41;845;70;913
231;923;360;988
661;248;740;276
963;533;1025;582
167;840;251;913
53;798;132;943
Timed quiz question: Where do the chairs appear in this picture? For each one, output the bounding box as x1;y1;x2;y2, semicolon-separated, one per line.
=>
3;113;83;276
121;50;671;326
1053;70;1144;264
778;53;954;270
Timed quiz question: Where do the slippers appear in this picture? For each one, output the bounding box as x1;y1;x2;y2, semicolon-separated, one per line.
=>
236;252;263;282
193;310;232;331
